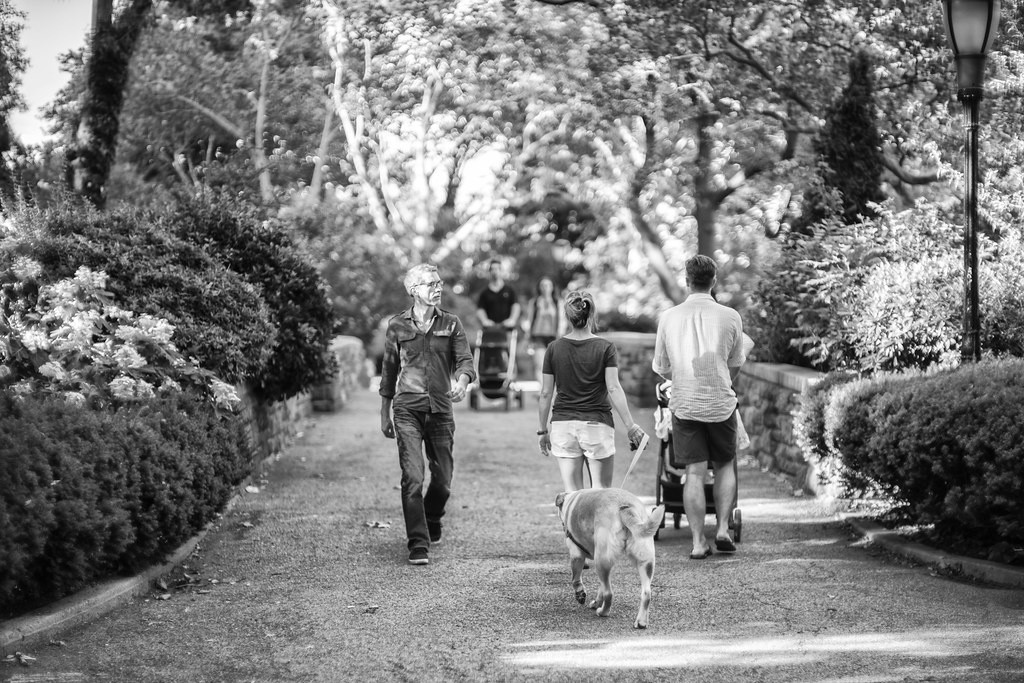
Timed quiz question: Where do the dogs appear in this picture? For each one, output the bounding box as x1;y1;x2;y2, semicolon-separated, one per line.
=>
554;488;665;628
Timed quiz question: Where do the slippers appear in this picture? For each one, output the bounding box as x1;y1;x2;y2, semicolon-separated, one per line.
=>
715;539;736;557
690;544;712;560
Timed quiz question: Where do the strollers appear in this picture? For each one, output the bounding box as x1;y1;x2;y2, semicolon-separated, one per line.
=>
653;384;747;544
468;325;528;411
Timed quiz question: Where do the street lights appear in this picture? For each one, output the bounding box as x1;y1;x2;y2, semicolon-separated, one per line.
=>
939;0;997;363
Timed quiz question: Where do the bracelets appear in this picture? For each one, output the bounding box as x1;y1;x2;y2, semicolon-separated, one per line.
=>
537;430;548;435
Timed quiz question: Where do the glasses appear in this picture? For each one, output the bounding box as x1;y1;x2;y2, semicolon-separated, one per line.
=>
410;280;444;289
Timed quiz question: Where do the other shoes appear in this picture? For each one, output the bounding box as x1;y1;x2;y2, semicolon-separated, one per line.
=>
409;547;428;565
428;523;442;545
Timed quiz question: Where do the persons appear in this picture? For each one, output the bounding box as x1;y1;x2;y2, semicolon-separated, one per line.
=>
524;275;565;381
653;256;746;559
477;261;521;328
538;291;649;568
380;263;476;564
